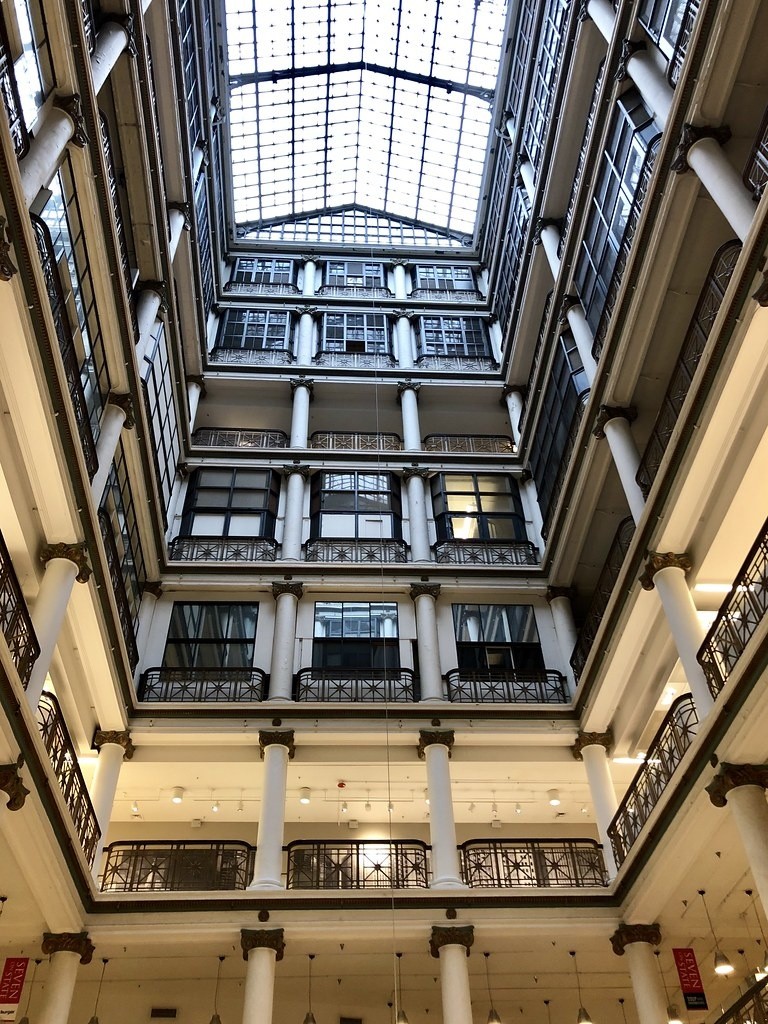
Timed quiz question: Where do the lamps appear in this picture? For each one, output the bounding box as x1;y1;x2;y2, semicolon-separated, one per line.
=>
492;803;497;813
483;953;501;1024
569;951;592;1024
395;953;406;1024
425;791;429;805
653;951;682;1024
19;959;43;1024
171;787;185;804
547;789;560;806
388;802;393;812
342;801;347;813
132;801;138;812
468;803;476;811
88;959;110;1024
514;803;521;815
299;787;310;805
699;891;734;975
303;955;316;1024
211;801;220;813
744;891;768;972
238;801;243;812
365;802;370;812
209;956;226;1024
